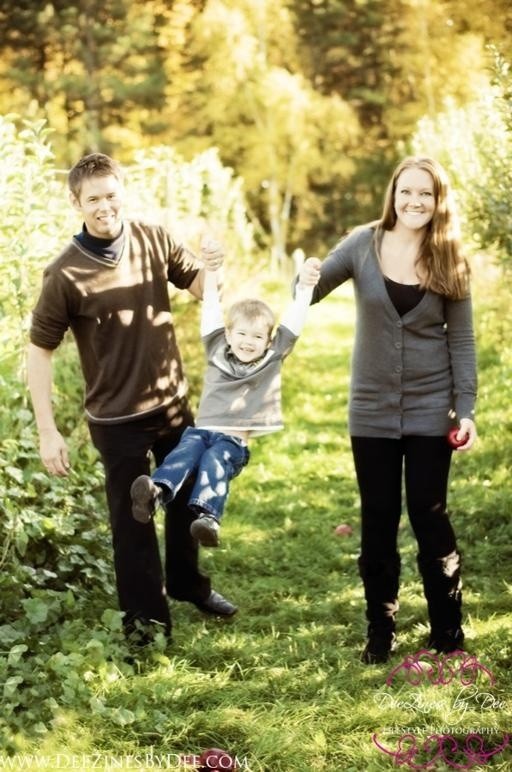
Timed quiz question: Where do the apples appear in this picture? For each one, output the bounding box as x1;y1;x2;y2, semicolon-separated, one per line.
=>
335;525;353;538
197;749;234;772
447;427;467;449
412;661;434;678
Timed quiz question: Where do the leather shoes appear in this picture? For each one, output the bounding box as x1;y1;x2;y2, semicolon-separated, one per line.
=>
191;590;238;618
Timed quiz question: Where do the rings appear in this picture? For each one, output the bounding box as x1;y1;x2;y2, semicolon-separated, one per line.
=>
213;251;219;258
215;258;220;264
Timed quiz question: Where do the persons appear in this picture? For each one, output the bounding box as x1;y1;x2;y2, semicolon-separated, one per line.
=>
22;152;240;666
295;158;479;667
130;234;325;549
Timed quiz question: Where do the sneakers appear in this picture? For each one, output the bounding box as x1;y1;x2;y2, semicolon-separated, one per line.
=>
189;511;222;547
359;637;396;665
129;473;161;524
427;629;465;653
